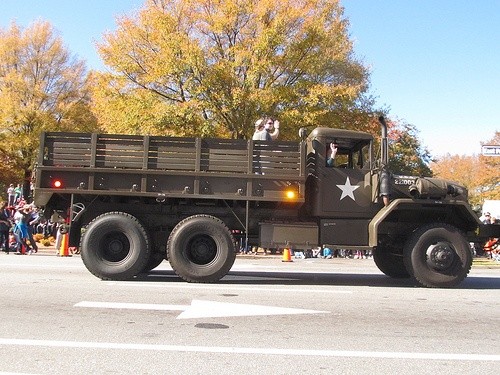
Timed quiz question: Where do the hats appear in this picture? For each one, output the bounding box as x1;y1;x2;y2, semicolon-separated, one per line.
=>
254;119;264;127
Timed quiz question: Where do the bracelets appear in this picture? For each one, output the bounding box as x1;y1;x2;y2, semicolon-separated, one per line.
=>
274;128;279;129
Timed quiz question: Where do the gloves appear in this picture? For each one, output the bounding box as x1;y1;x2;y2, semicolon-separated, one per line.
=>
273;120;280;129
330;143;338;159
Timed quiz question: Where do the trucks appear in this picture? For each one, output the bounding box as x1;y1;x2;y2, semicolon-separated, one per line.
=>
33;110;485;288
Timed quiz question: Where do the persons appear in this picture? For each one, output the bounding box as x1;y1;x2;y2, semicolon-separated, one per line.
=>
35;219;65;254
482;212;497;259
239;245;374;260
260;118;274;176
327;142;338;167
253;119;280;175
0;182;41;256
